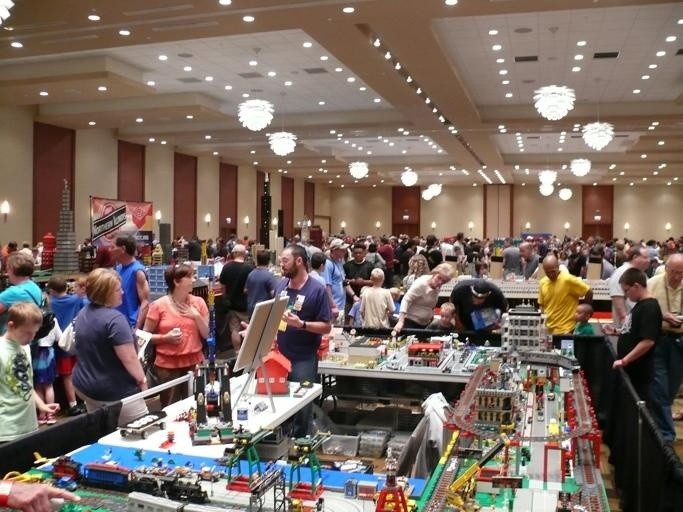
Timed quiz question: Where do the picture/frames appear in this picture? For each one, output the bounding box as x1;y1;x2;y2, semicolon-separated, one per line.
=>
97;375;322;459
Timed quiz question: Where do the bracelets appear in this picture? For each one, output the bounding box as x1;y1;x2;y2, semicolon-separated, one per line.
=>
619;357;626;365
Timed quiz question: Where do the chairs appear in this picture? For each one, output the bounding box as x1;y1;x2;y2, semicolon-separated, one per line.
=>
64;403;87;416
37;414;56;424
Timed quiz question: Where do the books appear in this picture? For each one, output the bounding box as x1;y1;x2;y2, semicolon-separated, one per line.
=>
316;422;362;457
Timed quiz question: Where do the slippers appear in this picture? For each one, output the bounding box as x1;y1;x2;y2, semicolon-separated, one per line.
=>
23;288;55;341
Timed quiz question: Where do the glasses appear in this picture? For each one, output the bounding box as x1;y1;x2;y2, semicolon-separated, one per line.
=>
237;98;299;158
531;58;614;151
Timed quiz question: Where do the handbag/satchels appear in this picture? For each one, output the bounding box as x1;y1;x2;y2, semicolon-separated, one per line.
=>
619;357;626;365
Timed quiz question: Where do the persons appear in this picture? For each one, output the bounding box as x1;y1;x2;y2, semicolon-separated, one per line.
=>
243;251;277;325
2;240;48;270
307;252;327;287
143;263;211;408
108;235;150;338
46;277;90;417
648;253;682;447
219;244;253;355
73;275;93;309
239;245;328;438
2;252;43;335
602;268;662;414
76;238;95;268
1;481;80;509
1;302;60;442
30;310;63;424
71;268;148;410
307;230;682;336
170;236;254;264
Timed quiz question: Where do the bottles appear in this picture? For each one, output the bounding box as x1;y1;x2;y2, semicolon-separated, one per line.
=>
330;239;351;252
471;279;492;298
231;244;245;253
